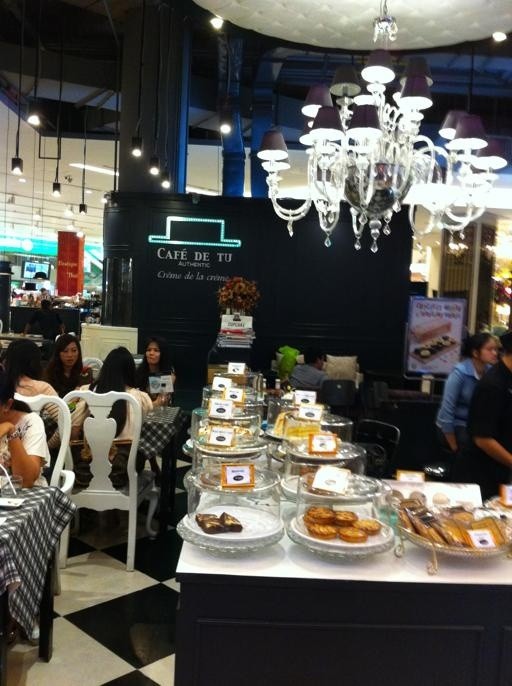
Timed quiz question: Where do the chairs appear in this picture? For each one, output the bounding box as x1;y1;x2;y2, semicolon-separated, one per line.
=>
354;417;401;478
14;393;78;495
82;355;102;380
58;390;145;572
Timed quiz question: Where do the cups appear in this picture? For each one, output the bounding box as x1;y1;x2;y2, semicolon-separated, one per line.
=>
1;476;22;498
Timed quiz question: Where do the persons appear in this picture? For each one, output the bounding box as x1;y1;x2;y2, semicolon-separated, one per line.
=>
460;328;512;501
1;334;176;488
12;287;102;340
435;332;499;480
289;349;329;390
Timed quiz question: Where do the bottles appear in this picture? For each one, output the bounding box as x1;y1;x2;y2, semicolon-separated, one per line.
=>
155;381;170;420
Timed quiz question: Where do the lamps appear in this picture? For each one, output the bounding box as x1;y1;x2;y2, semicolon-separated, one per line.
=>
220;18;234;133
79;101;88;214
133;3;145;158
52;77;62;198
110;81;119;207
211;14;225;31
149;2;160;177
12;76;23;174
258;0;508;253
161;88;172;188
28;51;40;126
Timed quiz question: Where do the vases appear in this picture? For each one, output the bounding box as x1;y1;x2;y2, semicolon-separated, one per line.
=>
229;311;244;328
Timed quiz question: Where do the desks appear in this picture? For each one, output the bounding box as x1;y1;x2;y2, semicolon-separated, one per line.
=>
139;402;184;542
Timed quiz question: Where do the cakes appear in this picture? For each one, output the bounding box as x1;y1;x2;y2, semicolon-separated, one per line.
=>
195;512;243;533
273;410;322;437
306;474;328;495
303;507;382;543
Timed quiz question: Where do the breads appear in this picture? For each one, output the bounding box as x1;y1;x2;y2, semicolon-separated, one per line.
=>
399;498;508;547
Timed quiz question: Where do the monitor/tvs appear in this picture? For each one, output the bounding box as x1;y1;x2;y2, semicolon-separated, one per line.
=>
21;261;50;280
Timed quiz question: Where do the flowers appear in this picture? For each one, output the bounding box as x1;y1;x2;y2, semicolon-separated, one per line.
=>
217;275;261;308
